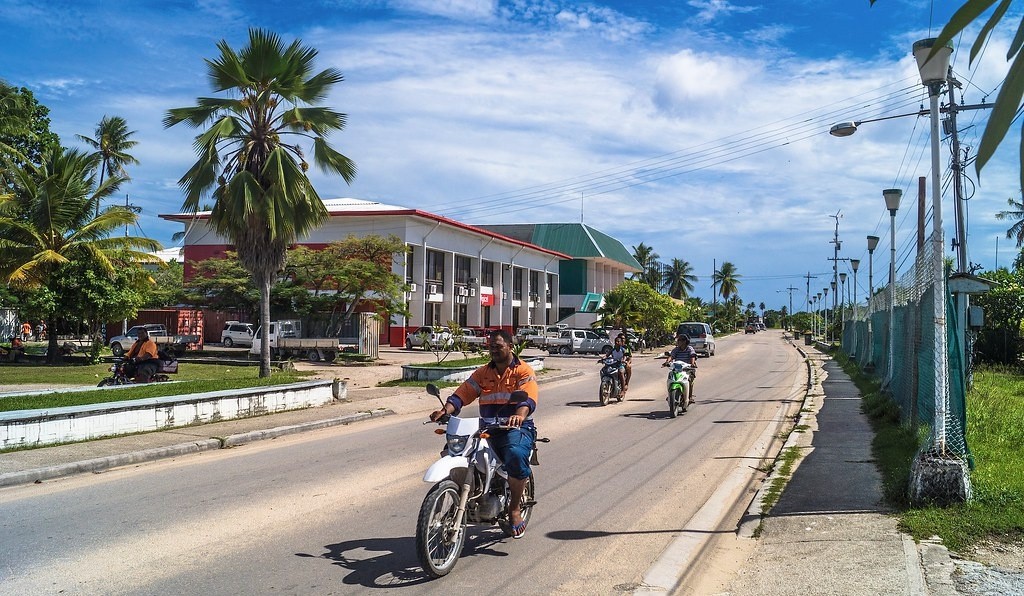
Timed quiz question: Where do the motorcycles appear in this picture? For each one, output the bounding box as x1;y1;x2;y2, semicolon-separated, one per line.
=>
596;357;629;407
661;350;698;419
416;384;553;580
97;360;171;389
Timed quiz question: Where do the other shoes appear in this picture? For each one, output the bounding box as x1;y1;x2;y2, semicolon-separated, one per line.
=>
43;337;45;339
690;398;695;403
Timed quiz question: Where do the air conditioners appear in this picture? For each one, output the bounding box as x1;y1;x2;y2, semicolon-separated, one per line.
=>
471;287;476;296
462;289;471;297
408;283;417;292
457;286;466;295
428;284;438;294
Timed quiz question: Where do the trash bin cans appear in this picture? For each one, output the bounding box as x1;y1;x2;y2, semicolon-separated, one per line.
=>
794;330;800;340
805;330;812;345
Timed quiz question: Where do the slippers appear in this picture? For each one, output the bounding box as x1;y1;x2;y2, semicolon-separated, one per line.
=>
509;517;525;539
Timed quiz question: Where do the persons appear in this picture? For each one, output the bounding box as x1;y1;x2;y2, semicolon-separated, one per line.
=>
430;329;537;539
122;327;158;383
10;320;47;355
596;334;698;404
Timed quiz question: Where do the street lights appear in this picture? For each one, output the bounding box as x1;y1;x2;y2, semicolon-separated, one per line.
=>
829;102;998;138
909;36;971;507
813;288;829;344
839;273;848;350
850;260;862;362
865;235;880;380
830;281;838;350
882;187;904;394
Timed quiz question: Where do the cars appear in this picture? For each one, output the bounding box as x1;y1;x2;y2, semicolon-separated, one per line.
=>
404;326;453;352
744;322;766;334
219;321;256;348
675;321;715;357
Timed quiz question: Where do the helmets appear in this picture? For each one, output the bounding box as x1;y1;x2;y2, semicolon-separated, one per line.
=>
40;320;44;324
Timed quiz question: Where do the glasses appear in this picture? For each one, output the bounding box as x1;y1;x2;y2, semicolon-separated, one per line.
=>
678;339;684;342
622;338;625;341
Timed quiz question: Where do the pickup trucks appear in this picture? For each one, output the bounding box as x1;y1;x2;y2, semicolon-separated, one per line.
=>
532;330;615;357
109;323;201;360
247;321;340;362
453;328;488;354
